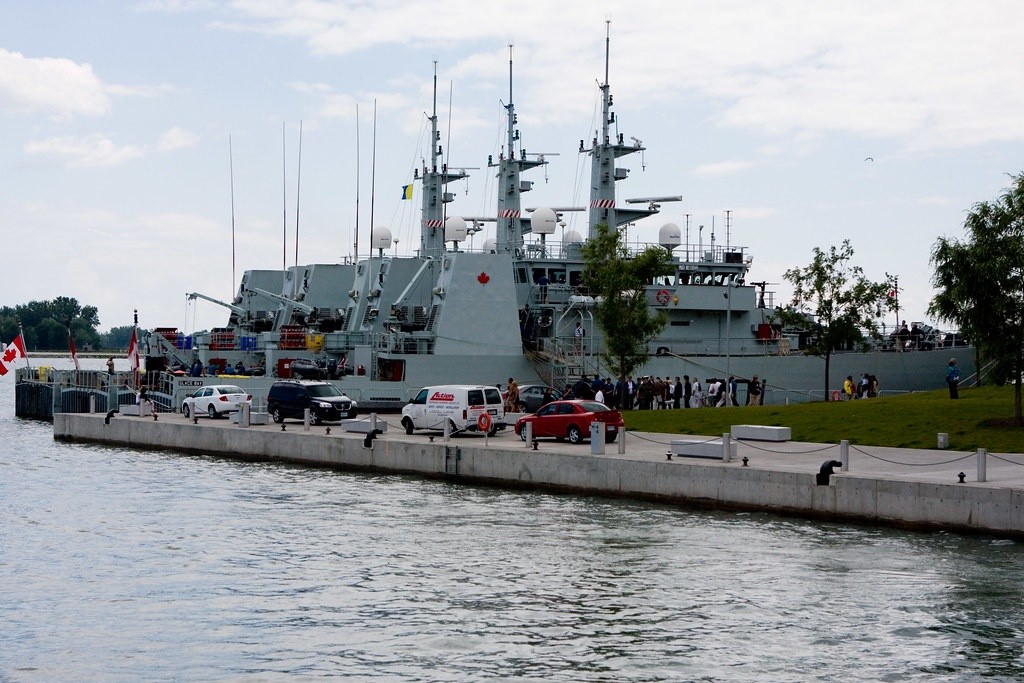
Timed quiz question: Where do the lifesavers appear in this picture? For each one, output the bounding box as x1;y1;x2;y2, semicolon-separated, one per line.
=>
832;391;840;401
655;290;670;304
478;412;491;431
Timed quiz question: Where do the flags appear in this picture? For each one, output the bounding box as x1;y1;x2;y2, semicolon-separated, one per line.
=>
402;184;412;200
0;334;25;376
69;330;81;370
129;326;139;371
888;290;894;298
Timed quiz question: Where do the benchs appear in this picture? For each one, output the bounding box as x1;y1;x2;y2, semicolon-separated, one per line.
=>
118;404;151;416
342;418;387;433
671;440;737;459
731;424;791;441
229;412;269;425
505;412;533;424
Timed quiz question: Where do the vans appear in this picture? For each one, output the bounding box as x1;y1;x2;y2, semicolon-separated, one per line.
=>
402;383;507;436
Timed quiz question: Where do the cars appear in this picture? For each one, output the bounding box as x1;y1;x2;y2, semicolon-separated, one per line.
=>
181;383;253;418
515;399;625;444
500;381;563;412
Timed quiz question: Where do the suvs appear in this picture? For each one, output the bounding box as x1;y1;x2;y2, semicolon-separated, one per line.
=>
266;376;360;426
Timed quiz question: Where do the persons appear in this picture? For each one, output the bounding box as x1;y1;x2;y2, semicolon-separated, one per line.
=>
841;373;879;400
496;377;524;414
521;304;532;351
574;322;586;357
562;374;740;412
106;356;115;381
540;387;556;414
173;358;246;377
744;376;761;406
531;273;553;305
889;320;945;353
136;386;158;420
945;357;962;400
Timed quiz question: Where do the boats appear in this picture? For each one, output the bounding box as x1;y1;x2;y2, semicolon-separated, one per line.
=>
569;294;596;309
289;360;328;376
596;295;607;308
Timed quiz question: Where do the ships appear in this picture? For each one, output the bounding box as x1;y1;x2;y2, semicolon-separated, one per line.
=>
16;17;984;422
